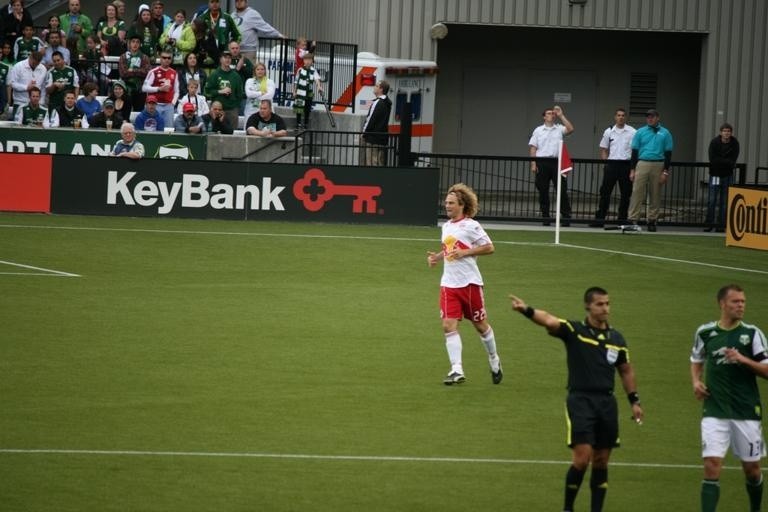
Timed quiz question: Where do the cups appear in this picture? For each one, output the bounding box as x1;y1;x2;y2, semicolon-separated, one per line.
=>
106;121;112;131
74;119;80;128
227;84;233;95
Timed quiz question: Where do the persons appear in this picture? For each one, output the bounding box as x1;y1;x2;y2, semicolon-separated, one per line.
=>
702;123;739;233
427;182;503;385
528;105;572;227
589;108;637;227
507;287;643;511
688;284;767;511
0;0;324;138
359;79;392;166
110;123;145;159
626;109;674;231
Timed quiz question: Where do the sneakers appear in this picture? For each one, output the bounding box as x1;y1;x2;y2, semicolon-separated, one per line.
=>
443;371;466;385
589;220;658;232
491;363;504;385
703;225;725;233
542;216;571;228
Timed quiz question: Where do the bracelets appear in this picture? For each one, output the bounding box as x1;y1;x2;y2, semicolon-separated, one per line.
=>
524;306;534;318
628;391;640;405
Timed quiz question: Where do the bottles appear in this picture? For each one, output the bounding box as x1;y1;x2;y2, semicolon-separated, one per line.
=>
38;114;43;129
31;75;36;87
208;121;213;134
620;225;641;231
78;115;81;127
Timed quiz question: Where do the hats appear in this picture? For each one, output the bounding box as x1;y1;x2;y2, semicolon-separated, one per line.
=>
112;79;127;92
103;99;115;109
645;108;659;116
145;95;158;104
183;103;195;113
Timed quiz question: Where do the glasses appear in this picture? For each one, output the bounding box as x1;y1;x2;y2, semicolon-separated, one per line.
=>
162;56;172;60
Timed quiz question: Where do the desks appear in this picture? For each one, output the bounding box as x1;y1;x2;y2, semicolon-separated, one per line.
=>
0;126;303;163
